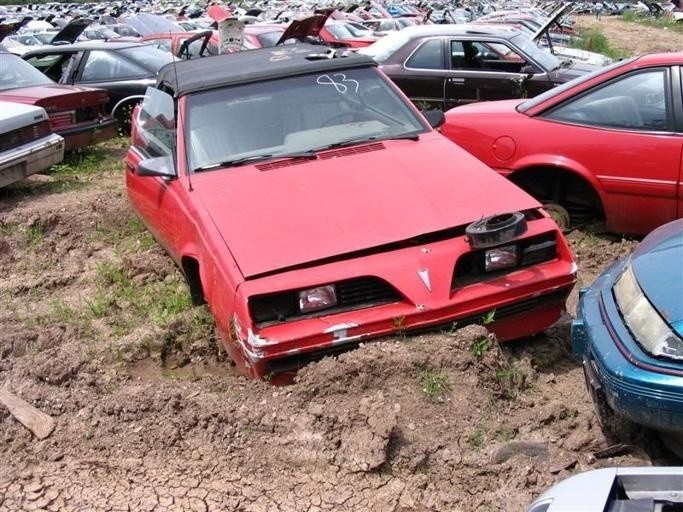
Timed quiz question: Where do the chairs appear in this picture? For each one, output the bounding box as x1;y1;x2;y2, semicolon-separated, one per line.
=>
284;82;354;136
582;95;645;128
467;46;491;71
187;102;278;172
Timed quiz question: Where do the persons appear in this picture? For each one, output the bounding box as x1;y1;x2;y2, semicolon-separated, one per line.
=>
593;0;604;22
